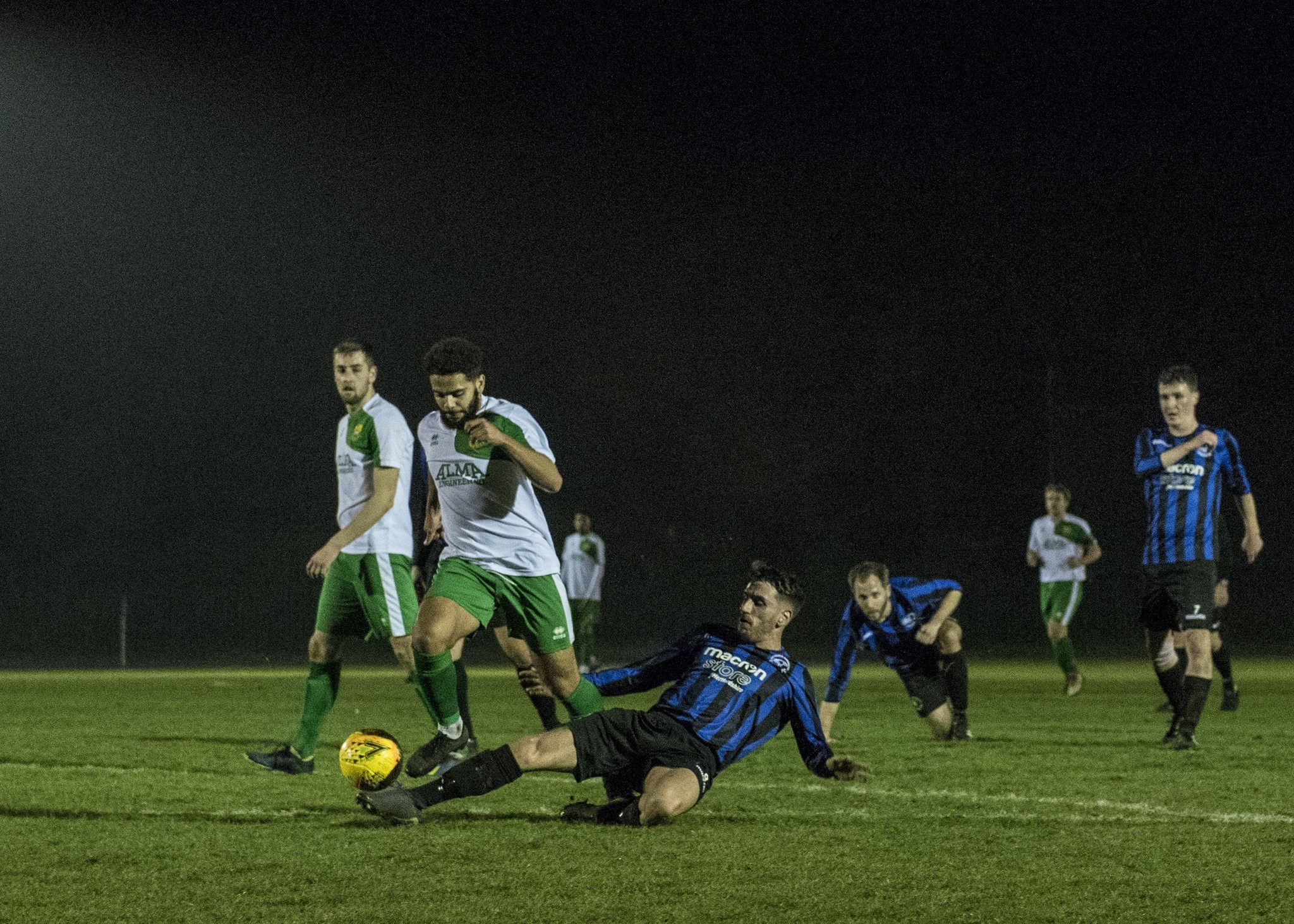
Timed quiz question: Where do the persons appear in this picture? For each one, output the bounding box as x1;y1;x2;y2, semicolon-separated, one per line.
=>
354;559;874;828
818;560;975;745
1027;484;1101;696
240;339;607;776
1133;365;1263;750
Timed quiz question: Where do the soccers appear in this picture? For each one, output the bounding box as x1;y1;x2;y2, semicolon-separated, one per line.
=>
338;729;403;790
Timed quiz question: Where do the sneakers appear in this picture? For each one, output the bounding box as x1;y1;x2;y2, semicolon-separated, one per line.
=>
356;785;420;824
558;797;629;824
425;739;479;776
405;725;469;778
241;743;313;777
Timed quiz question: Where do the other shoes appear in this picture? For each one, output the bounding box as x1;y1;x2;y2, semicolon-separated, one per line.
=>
1165;714;1185;742
947;713;968;743
1222;684;1240;712
1065;674;1083;696
1156;701;1173;713
603;774;634;800
1176;720;1198;748
